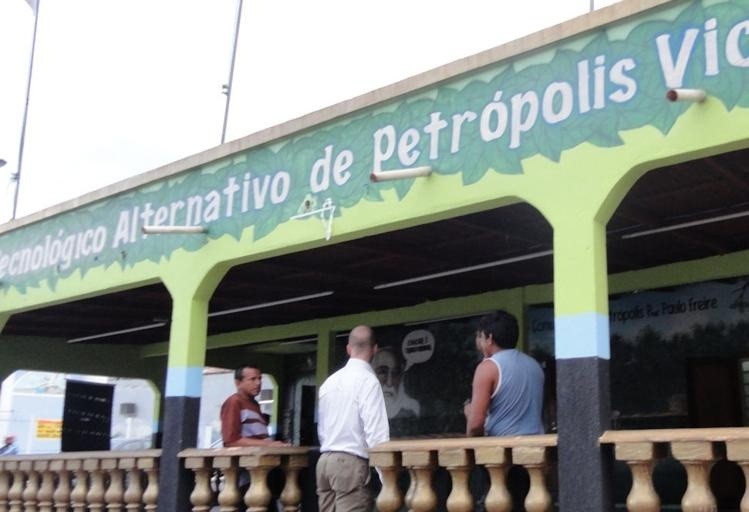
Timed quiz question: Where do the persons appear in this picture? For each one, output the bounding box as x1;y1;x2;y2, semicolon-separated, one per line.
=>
465;312;546;512
219;364;292;510
316;325;390;512
